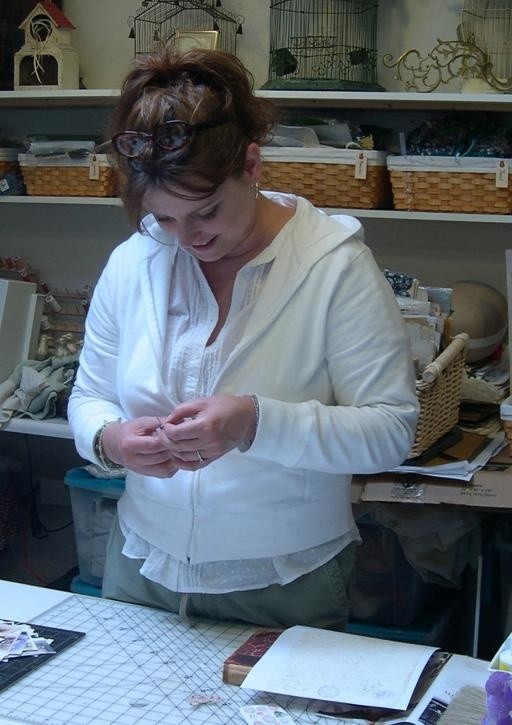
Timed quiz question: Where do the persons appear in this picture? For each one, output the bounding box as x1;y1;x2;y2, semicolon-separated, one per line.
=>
66;50;423;626
248;391;260;444
195;451;204;462
94;420;128;475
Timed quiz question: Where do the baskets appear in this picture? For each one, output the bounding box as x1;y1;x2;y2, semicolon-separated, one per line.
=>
0;146;25;178
18;152;119;197
386;153;512;214
259;146;389;209
500;394;512;457
406;333;469;460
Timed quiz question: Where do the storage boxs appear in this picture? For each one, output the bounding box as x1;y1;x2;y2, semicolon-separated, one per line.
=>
342;602;457;650
59;459;129;591
343;514;485;631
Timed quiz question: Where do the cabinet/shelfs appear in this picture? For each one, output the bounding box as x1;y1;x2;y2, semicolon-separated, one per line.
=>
1;89;512;229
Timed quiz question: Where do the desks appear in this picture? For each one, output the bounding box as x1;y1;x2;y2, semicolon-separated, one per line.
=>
0;408;511;544
2;574;511;722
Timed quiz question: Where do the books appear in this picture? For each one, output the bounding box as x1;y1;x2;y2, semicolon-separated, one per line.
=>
222;628;450;715
